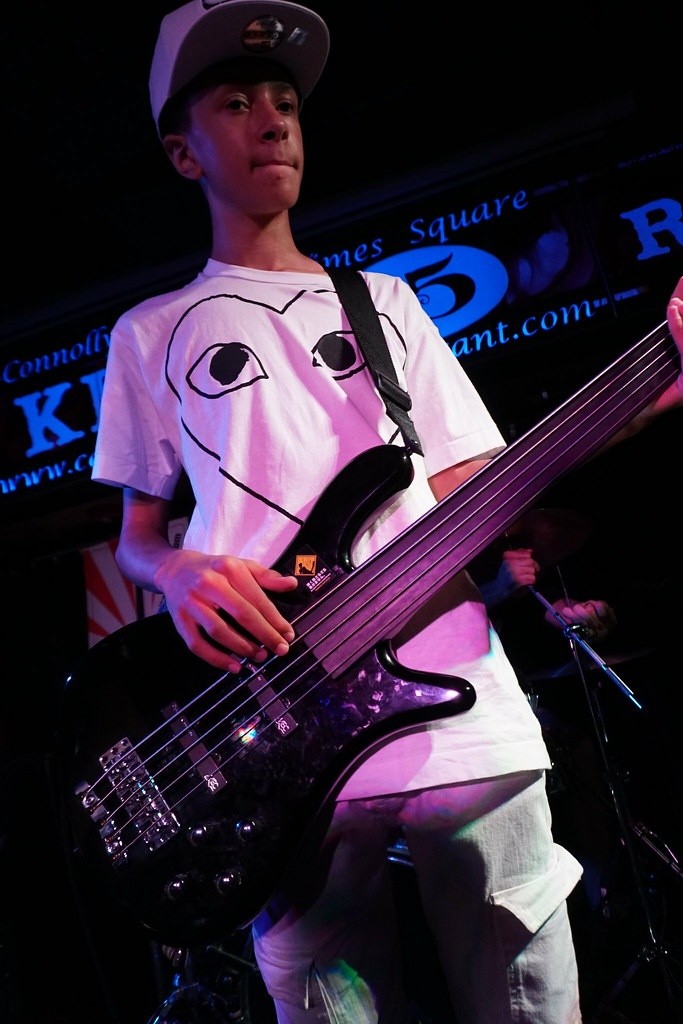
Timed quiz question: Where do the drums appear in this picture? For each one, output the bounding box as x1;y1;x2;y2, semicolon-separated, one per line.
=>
237;840;458;1024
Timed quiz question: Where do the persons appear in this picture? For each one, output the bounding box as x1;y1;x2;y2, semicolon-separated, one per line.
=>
472;509;615;734
93;0;683;1023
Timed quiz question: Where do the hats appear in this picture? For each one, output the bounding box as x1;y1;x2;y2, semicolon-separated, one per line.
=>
148;0;330;145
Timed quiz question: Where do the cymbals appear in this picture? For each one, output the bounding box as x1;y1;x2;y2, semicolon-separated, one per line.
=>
506;506;595;568
524;643;659;683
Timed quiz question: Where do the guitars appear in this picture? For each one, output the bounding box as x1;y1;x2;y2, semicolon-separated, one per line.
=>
50;278;683;950
